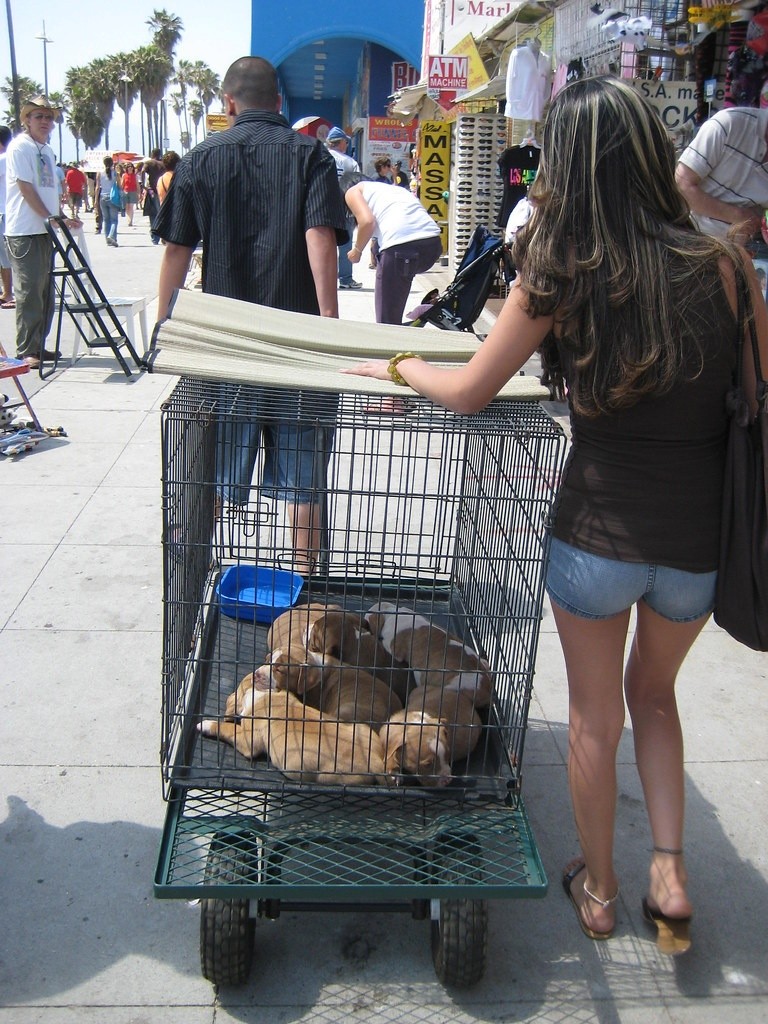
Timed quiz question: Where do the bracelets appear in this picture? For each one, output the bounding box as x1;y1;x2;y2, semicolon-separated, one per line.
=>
387;351;423;387
354;246;362;254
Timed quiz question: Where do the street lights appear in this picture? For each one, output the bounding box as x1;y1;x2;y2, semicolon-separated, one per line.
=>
34;19;55;144
119;69;133;152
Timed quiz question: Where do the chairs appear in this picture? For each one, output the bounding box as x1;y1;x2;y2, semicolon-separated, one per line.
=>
61;226;150;366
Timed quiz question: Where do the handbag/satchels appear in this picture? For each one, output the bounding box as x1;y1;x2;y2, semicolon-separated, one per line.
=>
110;184;121;207
143;193;158;216
714;396;768;652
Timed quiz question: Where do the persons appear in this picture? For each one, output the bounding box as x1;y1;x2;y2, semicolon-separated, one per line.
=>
673;77;768;242
5;94;84;367
55;156;170;247
0;125;16;309
150;56;340;574
156;151;181;208
338;74;768;956
368;155;410;269
339;171;443;326
502;184;537;289
323;126;362;289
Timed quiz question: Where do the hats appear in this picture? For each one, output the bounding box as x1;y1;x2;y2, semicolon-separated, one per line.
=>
19;96;62;130
70;161;80;169
327;126;351;143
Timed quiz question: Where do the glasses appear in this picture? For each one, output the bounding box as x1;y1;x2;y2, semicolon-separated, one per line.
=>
29;114;52;121
127;166;133;169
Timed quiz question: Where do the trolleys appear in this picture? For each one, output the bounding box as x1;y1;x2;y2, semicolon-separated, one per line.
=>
148;378;567;992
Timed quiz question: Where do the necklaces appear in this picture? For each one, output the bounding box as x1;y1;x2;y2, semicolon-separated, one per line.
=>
29;131;47;165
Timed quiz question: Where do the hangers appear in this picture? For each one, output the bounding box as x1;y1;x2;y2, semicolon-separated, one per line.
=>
519;128;543;149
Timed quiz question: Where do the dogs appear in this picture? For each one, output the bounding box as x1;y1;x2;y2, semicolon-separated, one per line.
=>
379;683;482;788
196;673;391;784
269;643;403;736
364;601;494;708
307;611;417;707
267;603;367;652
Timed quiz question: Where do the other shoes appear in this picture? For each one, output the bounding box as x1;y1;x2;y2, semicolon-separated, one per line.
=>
38;349;62;360
20;355;41;369
106;236;118;247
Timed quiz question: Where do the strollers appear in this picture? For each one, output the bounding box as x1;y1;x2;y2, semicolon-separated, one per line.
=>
400;220;514;333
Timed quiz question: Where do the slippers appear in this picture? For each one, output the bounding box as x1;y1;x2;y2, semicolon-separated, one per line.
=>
563;857;614;941
361;401;418;414
0;299;7;305
1;301;15;309
641;898;691;956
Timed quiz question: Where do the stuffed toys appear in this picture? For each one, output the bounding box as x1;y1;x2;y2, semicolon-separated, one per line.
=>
600;9;653;52
0;394;18;427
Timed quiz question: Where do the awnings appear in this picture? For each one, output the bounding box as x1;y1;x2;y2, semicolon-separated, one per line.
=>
474;0;569;47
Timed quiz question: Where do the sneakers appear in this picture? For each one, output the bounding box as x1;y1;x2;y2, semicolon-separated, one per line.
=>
339;280;362;289
